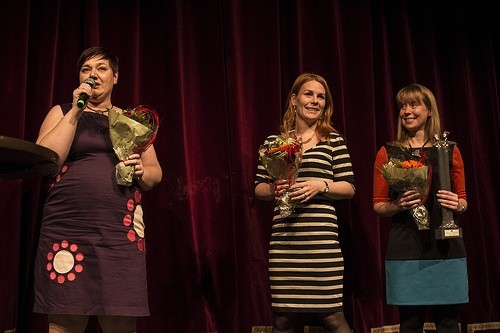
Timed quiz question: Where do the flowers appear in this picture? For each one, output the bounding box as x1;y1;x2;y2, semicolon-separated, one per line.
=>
258;130;303;220
107;104;159;187
378;143;433;231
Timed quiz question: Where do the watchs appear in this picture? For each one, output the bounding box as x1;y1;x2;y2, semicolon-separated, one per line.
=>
320;180;330;194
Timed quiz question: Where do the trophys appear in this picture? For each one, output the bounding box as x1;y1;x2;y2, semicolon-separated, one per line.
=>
433;132;463;240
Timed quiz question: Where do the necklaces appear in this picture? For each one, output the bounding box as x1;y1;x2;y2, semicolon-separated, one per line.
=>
405;135;430;158
86;104;113;114
293;131;315;144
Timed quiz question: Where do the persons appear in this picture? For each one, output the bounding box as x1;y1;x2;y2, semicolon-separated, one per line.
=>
254;73;358;333
33;47;162;333
373;83;467;333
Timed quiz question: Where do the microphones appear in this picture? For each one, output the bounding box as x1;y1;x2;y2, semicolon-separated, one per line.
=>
77;78;96;109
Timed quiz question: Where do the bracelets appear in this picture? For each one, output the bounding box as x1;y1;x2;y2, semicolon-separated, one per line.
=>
458;200;465;214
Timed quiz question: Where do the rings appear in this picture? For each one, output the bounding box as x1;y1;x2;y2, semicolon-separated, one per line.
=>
304;194;307;197
78;87;79;89
